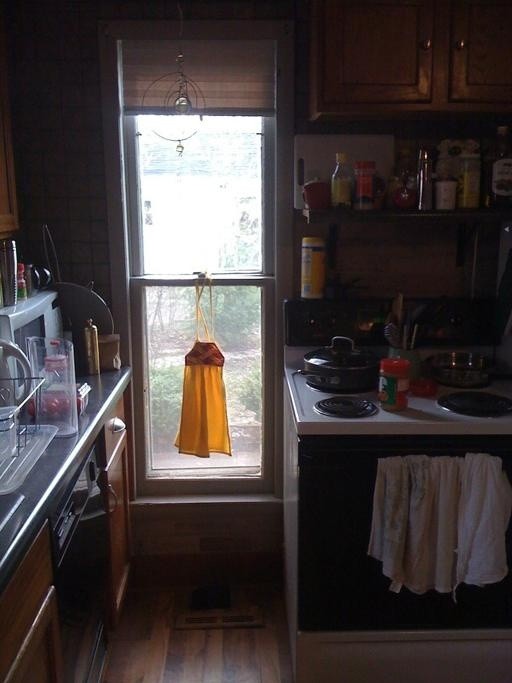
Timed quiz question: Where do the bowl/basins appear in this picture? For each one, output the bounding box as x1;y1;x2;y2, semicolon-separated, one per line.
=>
428;351;503;388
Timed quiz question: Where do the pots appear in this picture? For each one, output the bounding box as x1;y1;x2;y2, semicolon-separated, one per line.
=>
296;336;379;391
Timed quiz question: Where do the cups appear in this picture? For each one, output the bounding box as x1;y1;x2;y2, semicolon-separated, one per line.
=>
26;262;42;298
302;176;330;211
25;335;82;440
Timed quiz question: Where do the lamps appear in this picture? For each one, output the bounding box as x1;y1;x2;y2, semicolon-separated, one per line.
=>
142;0;206;156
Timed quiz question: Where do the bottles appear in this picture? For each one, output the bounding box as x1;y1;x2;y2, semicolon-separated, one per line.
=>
1;239;18;307
301;237;327;300
378;358;410;412
328;143;512;212
81;317;102;376
16;263;26;300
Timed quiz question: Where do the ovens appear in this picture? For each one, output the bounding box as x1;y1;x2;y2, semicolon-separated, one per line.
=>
283;380;510;683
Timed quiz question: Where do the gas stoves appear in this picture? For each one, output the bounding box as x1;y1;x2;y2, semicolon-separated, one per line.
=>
285;362;512;435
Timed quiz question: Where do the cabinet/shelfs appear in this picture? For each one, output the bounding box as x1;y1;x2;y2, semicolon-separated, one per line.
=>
105;381;136;629
308;0;512;124
0;517;66;683
0;0;21;241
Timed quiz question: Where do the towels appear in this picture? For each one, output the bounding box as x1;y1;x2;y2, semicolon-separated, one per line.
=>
366;453;512;603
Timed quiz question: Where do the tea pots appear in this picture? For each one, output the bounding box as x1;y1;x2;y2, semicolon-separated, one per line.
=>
0;338;34;407
